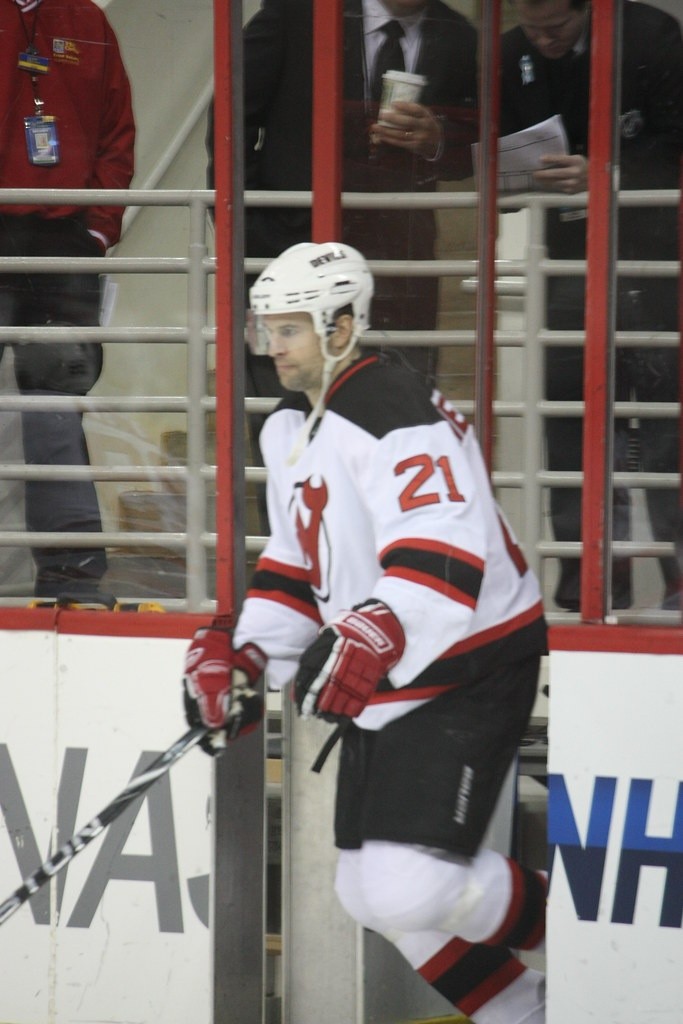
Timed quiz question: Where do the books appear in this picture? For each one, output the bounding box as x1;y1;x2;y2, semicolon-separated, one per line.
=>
470;114;571;194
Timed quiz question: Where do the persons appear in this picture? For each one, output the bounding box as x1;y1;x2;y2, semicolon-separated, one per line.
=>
0;0;137;610
492;0;683;610
208;0;482;387
182;242;550;1024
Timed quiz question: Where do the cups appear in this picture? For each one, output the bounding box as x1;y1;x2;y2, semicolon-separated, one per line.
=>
377;69;429;131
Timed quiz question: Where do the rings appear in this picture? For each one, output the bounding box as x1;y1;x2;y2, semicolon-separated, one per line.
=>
405;130;415;141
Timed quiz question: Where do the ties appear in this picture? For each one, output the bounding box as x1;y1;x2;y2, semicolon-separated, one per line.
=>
370;18;409;104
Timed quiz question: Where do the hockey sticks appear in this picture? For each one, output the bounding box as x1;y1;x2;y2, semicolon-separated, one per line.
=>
0;685;262;927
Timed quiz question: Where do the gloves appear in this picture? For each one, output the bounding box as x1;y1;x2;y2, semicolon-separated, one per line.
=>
179;624;271;757
292;597;406;730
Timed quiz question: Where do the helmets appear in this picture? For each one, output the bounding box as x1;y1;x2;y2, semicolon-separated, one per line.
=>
248;241;372;361
